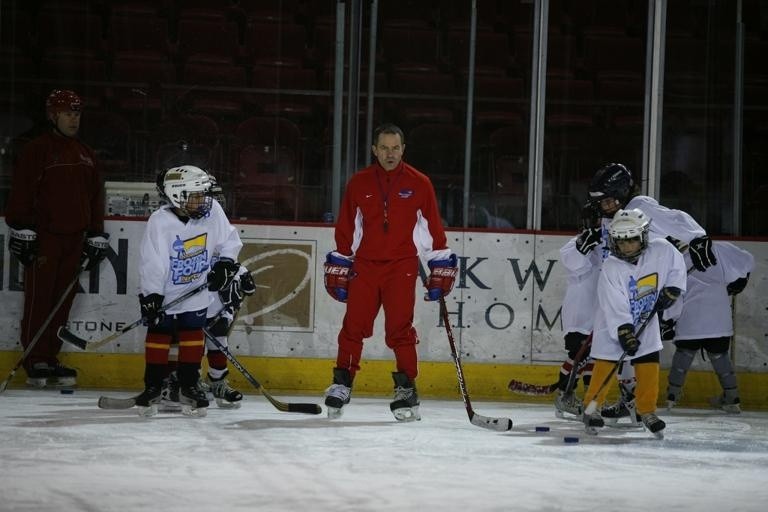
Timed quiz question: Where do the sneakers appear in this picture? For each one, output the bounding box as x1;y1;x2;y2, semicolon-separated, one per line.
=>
48;358;77;377
23;361;50;378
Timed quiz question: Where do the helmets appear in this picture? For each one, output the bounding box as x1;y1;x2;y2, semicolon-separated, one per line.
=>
45;88;86;120
577;162;653;264
156;165;227;220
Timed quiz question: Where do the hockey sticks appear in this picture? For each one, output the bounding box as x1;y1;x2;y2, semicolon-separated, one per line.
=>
202;324;321;415
58;280;208;353
0;227;119;393
563;332;592;403
509;359;590;394
578;305;655;430
439;296;512;430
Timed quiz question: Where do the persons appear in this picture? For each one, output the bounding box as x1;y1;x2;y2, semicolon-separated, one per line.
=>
579;206;688;432
322;124;457;413
4;88;106;381
587;162;717;417
660;239;754;405
135;165;244;410
154;168;256;402
554;199;603;414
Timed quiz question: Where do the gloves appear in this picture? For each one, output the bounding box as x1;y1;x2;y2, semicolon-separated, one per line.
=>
658;318;677;341
564;332;591;360
324;249;355;303
617;323;641;356
689;235;717;272
80;231;110;271
206;257;256;329
423;254;458;301
727;272;750;296
657;286;681;311
8;229;39;267
140;294;167;328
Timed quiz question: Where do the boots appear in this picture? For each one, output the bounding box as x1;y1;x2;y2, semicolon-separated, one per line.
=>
136;364;242;407
325;367;353;408
709;386;739;407
555;382;666;433
390;371;420;411
666;384;683;402
